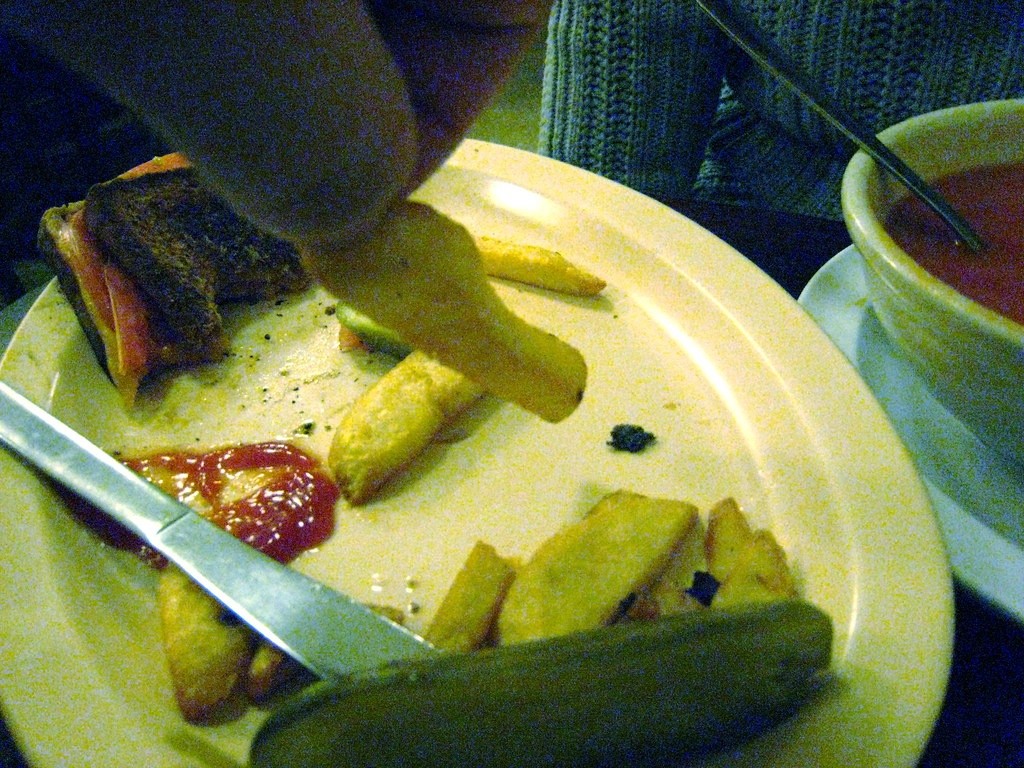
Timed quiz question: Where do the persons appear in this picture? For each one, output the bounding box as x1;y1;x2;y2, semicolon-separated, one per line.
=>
0;0;558;240
538;0;1024;299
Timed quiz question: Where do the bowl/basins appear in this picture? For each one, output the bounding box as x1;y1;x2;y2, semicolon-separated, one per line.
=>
839;99;1024;468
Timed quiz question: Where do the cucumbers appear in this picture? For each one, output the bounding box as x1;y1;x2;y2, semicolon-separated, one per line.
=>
246;598;831;768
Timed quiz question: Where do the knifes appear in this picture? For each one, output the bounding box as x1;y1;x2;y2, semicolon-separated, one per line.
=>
0;381;438;683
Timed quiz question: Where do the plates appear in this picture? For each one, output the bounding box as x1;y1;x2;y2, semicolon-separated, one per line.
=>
796;240;1024;628
0;140;952;761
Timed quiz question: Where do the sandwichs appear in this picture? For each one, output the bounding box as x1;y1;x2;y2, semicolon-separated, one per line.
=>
36;155;309;414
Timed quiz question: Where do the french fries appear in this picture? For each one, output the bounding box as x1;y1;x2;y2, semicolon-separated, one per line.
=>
157;194;794;723
